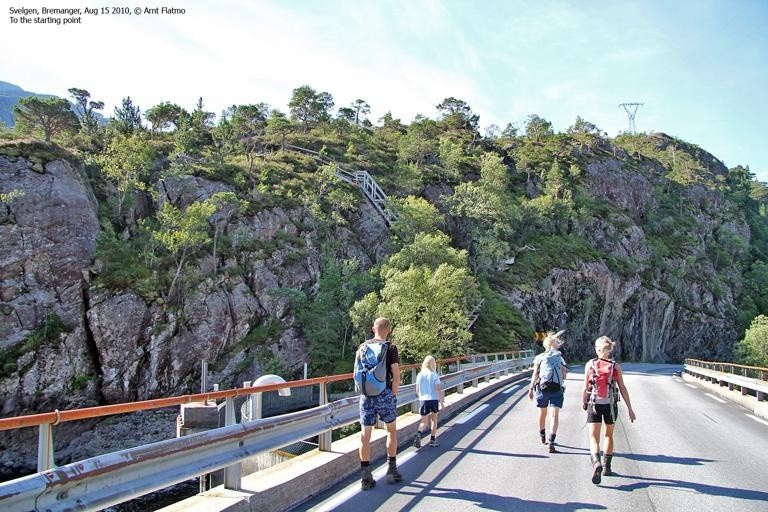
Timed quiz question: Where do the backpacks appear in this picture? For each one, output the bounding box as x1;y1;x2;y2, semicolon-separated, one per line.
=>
353;342;391;396
586;357;617;422
538;350;563;392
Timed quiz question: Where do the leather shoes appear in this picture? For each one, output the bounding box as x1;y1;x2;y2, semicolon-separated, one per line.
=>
360;479;377;491
386;471;402;484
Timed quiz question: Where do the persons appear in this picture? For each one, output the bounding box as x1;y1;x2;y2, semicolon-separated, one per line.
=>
414;355;445;448
582;335;636;485
352;317;403;491
529;336;566;453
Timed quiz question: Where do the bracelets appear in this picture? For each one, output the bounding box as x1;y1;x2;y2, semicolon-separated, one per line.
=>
530;388;533;390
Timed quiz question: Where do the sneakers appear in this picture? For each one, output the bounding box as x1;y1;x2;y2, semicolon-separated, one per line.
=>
429;440;440;447
540;437;546;444
548;444;555;453
413;433;421;448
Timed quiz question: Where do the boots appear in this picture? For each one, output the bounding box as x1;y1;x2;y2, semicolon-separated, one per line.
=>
591;452;603;485
602;453;613;476
